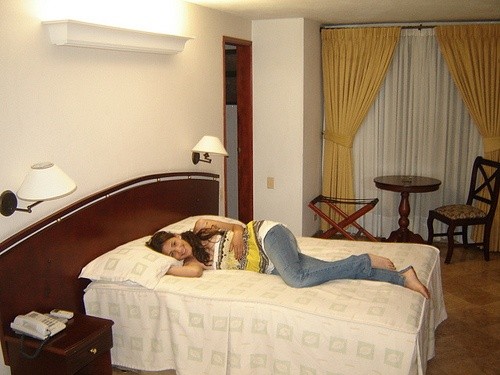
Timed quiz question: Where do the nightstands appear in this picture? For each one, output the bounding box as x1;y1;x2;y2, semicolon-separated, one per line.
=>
5;294;115;375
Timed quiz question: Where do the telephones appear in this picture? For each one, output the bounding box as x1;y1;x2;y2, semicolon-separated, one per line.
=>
10;310;67;340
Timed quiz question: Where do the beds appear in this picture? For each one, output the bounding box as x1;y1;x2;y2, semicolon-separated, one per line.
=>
0;173;449;375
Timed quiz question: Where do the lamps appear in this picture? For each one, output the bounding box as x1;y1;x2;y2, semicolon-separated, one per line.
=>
0;162;77;217
42;19;192;56
192;135;229;167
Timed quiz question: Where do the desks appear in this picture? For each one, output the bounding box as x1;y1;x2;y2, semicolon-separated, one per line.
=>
372;174;442;244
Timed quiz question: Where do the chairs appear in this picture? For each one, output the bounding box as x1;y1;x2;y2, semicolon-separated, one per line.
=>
428;156;500;264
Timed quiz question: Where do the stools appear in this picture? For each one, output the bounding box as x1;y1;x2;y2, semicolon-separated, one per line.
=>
308;192;380;241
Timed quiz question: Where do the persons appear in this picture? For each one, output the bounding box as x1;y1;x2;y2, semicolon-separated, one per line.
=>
145;218;430;299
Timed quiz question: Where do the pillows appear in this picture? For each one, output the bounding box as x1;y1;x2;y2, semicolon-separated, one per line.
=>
77;234;183;289
159;214;242;236
